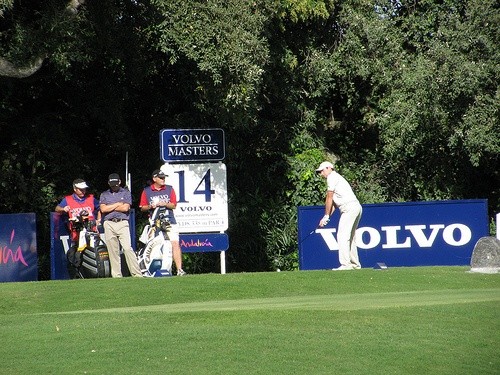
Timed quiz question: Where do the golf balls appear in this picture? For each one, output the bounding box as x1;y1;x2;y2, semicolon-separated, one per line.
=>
277;269;280;272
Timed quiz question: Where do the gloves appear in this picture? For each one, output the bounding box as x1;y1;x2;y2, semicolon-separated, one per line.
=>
319;215;330;227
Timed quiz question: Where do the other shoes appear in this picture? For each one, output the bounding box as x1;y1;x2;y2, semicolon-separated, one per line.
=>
332;264;354;270
353;264;361;269
177;268;187;276
132;273;143;277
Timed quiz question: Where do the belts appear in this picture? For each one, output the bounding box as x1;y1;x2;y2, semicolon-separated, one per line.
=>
108;217;128;222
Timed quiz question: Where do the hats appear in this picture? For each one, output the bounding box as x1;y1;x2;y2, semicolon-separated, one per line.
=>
73;181;89;188
153;170;168;178
315;162;334;176
109;177;119;186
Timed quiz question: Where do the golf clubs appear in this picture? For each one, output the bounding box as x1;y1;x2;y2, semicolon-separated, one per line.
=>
274;217;332;263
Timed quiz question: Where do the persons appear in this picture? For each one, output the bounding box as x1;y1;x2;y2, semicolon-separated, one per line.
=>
139;170;186;276
55;179;101;279
316;161;363;271
99;174;143;278
